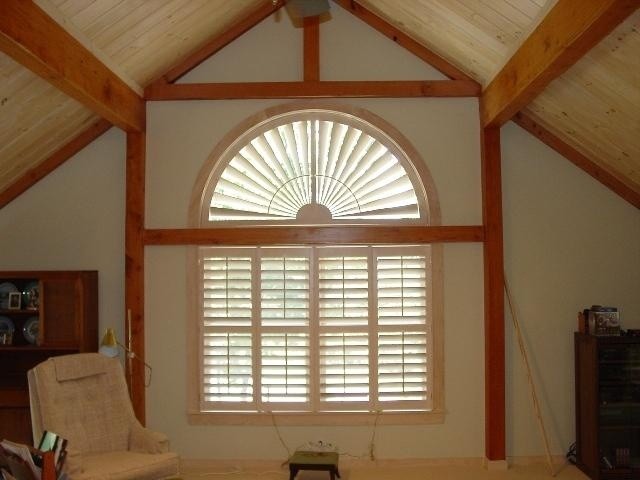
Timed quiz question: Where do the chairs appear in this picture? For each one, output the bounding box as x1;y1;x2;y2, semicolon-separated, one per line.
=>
27;353;180;480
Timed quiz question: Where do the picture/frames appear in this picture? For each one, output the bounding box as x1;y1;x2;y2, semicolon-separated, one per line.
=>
8;292;21;310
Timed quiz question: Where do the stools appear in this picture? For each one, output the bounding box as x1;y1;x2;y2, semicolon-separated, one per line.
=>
287;450;340;480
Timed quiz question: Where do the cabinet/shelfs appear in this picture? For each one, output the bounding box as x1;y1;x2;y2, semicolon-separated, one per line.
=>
0;269;99;448
573;331;640;480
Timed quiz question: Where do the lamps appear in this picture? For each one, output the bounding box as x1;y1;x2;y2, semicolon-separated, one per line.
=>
285;0;332;20
100;308;155;402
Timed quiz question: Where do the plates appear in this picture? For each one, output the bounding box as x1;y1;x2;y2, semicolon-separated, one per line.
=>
0;281;40;342
